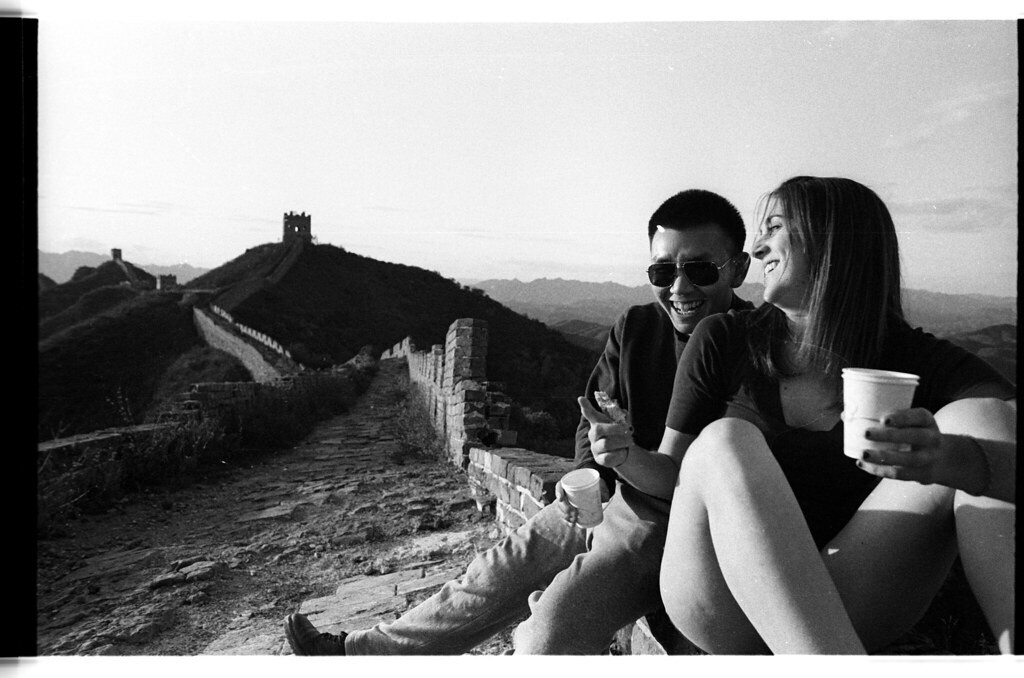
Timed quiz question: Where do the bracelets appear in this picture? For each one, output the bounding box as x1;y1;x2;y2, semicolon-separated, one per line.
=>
612;446;630;468
968;438;992;498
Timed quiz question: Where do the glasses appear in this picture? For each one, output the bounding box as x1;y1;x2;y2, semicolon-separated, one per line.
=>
646;256;734;288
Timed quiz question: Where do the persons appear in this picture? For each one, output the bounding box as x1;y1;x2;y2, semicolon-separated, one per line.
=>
285;189;759;656
578;176;1017;655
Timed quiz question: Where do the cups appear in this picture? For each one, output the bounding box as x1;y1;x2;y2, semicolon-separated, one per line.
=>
842;369;919;458
561;468;604;528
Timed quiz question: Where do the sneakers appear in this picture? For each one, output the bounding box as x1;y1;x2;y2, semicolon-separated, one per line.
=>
283;613;347;656
